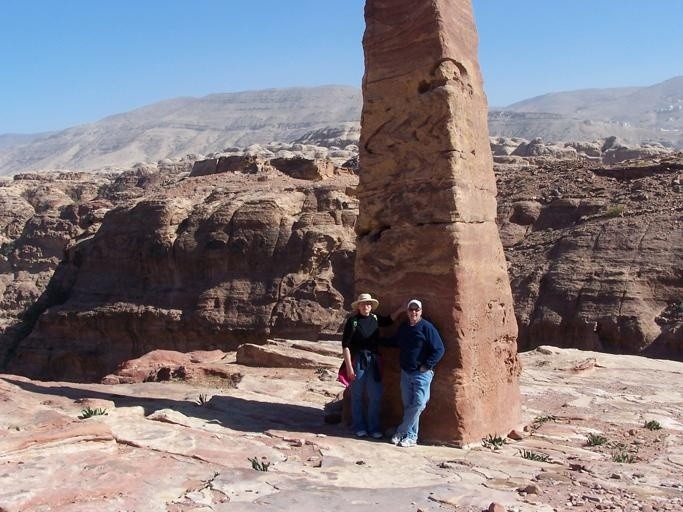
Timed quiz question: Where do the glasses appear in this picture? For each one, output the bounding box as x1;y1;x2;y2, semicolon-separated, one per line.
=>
409;308;419;312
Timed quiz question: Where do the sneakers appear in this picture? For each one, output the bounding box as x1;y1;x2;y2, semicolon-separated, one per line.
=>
356;431;368;437
369;432;383;438
399;437;417;447
391;433;403;444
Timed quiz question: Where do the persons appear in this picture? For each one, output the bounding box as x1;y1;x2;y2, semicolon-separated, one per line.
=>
379;299;445;448
342;293;411;438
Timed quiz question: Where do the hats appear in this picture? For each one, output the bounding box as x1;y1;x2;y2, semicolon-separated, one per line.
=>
408;300;422;308
351;294;379;310
